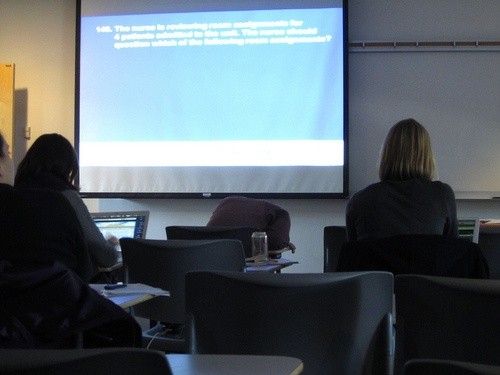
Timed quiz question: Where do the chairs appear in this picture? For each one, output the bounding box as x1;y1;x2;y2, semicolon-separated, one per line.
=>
0;226;500;375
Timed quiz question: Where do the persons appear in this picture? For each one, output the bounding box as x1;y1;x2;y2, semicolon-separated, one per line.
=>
0;134;60;266
346;118;458;240
13;133;120;268
205;195;295;251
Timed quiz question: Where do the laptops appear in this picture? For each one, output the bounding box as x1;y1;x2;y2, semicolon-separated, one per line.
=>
90;210;149;272
458;216;480;245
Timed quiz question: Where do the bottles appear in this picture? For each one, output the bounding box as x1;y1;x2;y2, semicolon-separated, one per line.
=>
252;232;268;263
